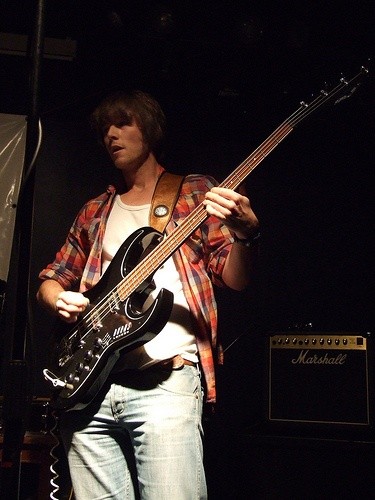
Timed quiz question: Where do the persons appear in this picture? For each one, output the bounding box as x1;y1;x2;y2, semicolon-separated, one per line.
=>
34;84;262;500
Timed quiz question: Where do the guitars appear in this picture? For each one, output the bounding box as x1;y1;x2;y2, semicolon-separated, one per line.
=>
43;56;375;414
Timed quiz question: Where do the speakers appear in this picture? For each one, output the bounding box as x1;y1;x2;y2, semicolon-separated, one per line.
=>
268;335;370;427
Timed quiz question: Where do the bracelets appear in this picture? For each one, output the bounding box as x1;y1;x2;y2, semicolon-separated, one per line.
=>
233;233;262;246
